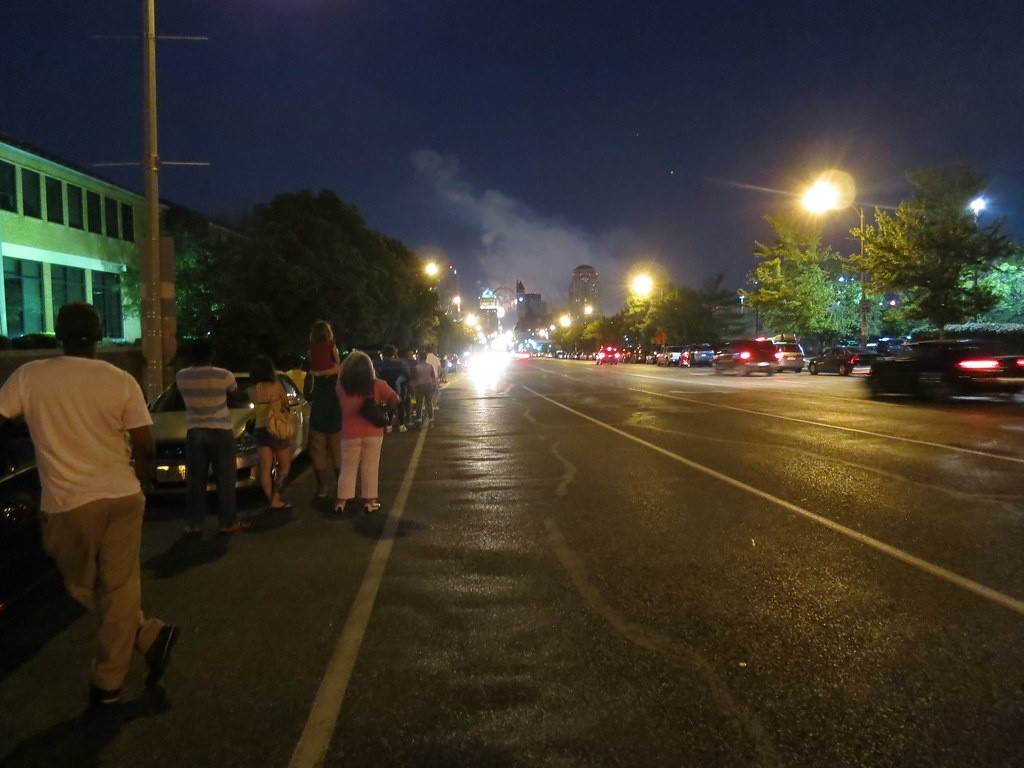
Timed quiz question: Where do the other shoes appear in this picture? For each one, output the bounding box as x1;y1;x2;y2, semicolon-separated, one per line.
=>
334;499;345;512
364;499;381;513
399;424;407;433
408;422;413;425
222;520;253;533
386;426;392;433
417;418;422;422
145;623;181;686
184;525;201;537
315;490;326;499
429;416;434;421
92;683;122;704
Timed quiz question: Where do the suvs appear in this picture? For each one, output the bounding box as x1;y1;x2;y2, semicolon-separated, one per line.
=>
595;347;619;366
656;345;683;366
678;343;714;368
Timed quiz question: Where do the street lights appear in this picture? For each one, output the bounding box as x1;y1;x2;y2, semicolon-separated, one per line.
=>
631;275;666;353
800;182;868;349
584;305;605;347
969;197;985;322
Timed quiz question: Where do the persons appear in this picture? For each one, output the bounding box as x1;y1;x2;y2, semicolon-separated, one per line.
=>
310;320;342;376
0;303;181;706
377;345;460;433
246;355;295;510
176;338;253;533
332;352;400;513
303;372;341;498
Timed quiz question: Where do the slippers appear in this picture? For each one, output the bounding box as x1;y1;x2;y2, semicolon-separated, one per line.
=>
268;503;292;511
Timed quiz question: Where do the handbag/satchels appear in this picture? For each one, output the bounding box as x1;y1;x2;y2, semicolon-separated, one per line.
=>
303;372;314;402
265;400;298;439
360;397;389;426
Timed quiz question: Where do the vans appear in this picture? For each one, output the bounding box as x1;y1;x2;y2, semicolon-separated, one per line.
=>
716;339;779;377
772;341;805;373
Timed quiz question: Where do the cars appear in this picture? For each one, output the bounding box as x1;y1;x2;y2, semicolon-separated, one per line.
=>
126;372;313;494
808;347;877;377
866;338;1024;404
532;351;658;365
